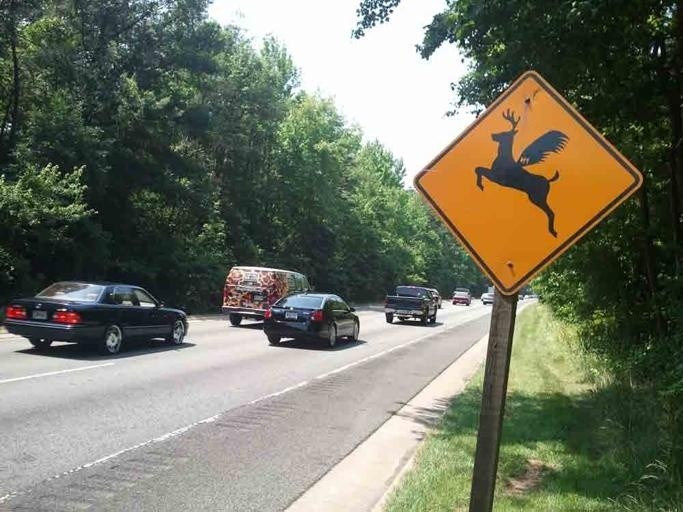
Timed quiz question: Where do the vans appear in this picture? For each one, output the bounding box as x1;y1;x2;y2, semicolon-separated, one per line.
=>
221;265;311;325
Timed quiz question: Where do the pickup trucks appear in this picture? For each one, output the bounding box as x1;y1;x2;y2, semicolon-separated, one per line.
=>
384;286;438;325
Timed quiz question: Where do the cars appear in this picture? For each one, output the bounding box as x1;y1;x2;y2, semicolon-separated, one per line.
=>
263;290;359;349
2;280;188;356
452;283;526;306
428;289;441;309
